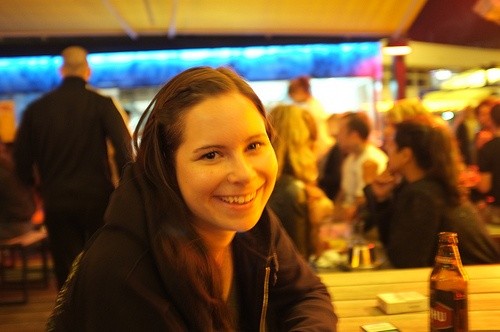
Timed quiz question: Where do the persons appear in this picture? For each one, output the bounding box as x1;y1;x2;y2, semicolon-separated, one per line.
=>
0;47;132;295
265;75;500;272
46;66;338;332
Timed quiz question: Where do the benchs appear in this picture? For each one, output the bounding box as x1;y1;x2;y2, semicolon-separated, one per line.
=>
0;230;48;304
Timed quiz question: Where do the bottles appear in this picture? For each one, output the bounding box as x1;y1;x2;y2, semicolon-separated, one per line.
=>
429;231;469;332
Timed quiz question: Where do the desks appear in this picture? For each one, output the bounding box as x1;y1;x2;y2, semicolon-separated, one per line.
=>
319;264;500;332
